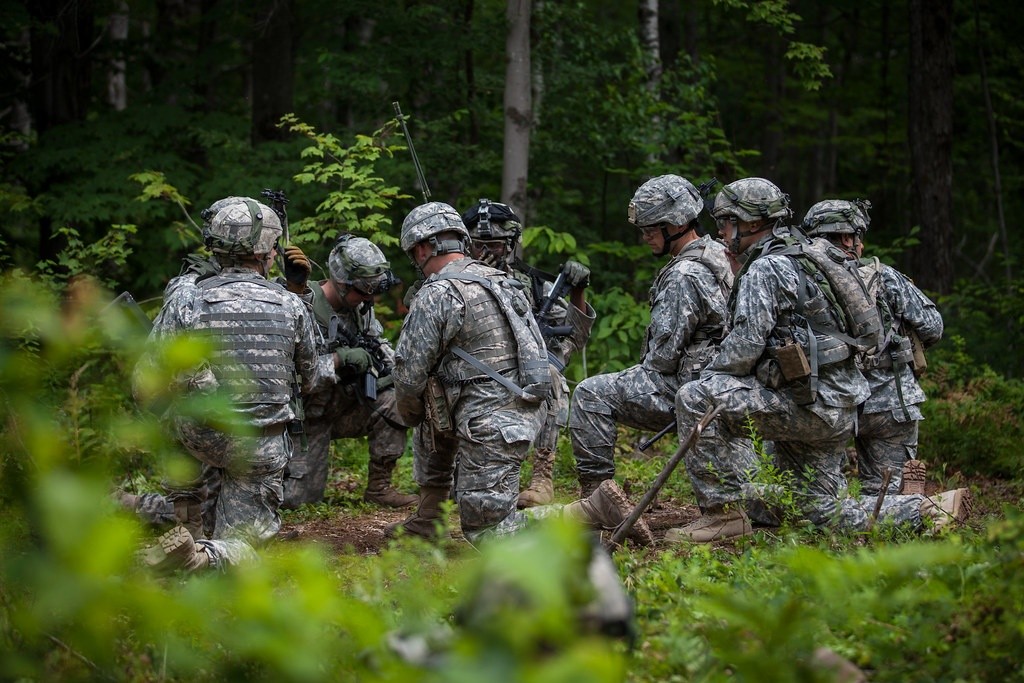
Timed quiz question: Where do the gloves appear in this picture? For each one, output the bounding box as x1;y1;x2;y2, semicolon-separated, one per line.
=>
335;346;374;376
478;250;501;269
559;261;590;288
283;246;312;294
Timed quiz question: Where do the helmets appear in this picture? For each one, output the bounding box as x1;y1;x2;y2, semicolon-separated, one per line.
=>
401;201;473;252
627;174;704;227
713;178;789;222
469;203;517;242
801;200;866;236
328;238;392;285
203;197;260;231
208;199;283;255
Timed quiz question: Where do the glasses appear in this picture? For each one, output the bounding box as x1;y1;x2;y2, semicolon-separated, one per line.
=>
716;216;731;231
640;226;660;237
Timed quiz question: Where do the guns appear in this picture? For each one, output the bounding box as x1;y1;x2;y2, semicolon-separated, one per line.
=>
638;407;678;453
534;267;579;372
98;292;155;343
327;315;390;403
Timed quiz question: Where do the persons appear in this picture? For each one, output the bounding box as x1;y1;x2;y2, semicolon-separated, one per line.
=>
381;205;655;548
108;198;323;577
569;174;781;523
280;238;420;509
761;200;943;494
402;200;597;507
666;178;972;546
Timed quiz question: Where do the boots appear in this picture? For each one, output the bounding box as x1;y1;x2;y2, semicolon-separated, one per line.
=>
563;479;655;550
384;486;452;543
174;501;207;541
919;487;974;535
663;502;754;546
889;460;926;496
517;447;555;510
581;476;600;499
364;461;420;509
100;477;140;520
128;527;209;579
